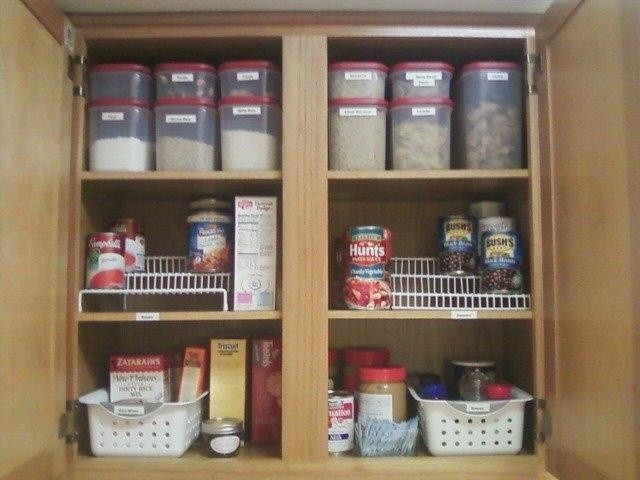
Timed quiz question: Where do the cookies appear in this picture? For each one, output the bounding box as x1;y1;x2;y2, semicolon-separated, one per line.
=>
266;370;282;398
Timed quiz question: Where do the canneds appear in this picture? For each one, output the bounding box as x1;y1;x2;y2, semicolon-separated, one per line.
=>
187;197;232;272
437;200;524;308
84;231;127;288
450;356;499;402
326;348;407;457
110;218;145;273
329;226;394;310
202;417;245;457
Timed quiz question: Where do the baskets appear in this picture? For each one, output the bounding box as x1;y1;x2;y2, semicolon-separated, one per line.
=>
406;375;534;456
78;387;210;458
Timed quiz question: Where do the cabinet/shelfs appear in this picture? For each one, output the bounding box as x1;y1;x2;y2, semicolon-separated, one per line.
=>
0;1;640;479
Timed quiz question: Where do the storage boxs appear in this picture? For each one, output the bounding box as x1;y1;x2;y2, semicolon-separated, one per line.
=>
86;59;281;171
330;60;524;171
